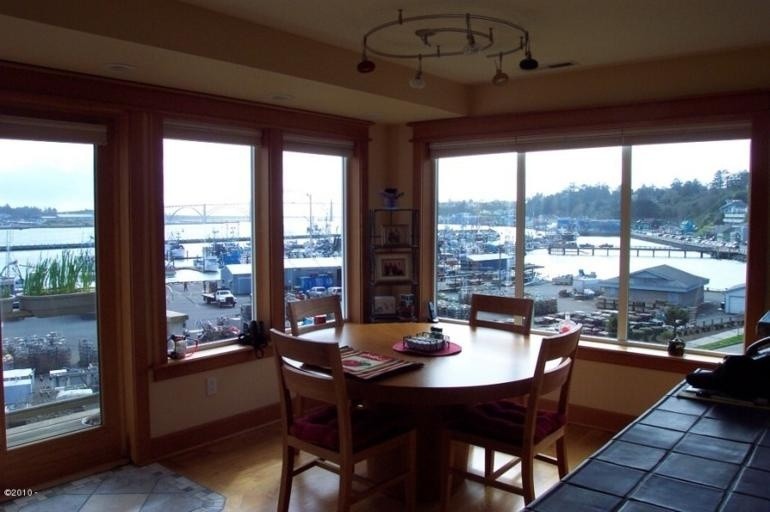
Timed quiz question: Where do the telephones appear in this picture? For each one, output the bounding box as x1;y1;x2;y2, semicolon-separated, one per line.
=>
428;302;439;323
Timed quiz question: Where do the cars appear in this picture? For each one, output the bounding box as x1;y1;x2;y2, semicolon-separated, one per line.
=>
308;287;325;297
327;286;342;297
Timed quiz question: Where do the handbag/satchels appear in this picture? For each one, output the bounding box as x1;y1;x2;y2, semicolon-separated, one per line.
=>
242;321;269;348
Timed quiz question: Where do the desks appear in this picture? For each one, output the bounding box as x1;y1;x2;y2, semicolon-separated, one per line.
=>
281;323;562;511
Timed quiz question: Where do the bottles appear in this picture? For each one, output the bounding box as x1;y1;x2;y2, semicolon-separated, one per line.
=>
667;338;685;356
431;327;442;335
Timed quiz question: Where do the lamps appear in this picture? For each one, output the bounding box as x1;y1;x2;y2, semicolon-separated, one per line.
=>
357;9;538;89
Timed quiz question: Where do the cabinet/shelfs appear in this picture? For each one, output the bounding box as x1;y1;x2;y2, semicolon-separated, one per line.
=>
368;208;419;323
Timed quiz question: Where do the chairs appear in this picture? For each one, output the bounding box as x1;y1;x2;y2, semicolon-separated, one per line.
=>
442;323;582;507
468;293;534;407
270;328;417;511
287;295;344;456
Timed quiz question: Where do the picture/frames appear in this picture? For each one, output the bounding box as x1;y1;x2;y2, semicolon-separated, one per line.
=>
374;253;412;283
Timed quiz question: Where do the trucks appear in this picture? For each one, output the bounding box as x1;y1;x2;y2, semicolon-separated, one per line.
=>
202;287;237;308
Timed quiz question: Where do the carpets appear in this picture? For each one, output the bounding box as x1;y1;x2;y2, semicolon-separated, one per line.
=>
0;461;229;512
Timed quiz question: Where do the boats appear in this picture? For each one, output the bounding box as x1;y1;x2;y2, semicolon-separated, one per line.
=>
194;222;252;273
510;264;544;286
438;229;513;293
165;230;185;259
522;223;577;251
285;237;341;258
164;261;175;278
